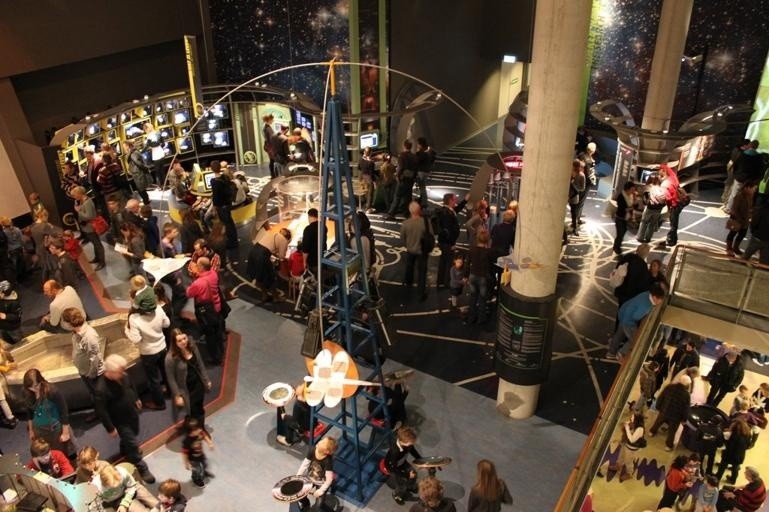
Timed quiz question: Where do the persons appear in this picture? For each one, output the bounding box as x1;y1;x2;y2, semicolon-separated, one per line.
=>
249;209;328;303
562;127;598;242
345;212;378;322
724;139;769;266
467;459;513;511
1;140;249;427
263;114;313;178
359;136;436;221
277;381;455;512
400;201;435;303
20;328;213;511
436;192;518;318
599;333;768;510
612;164;691;253
606;243;667;360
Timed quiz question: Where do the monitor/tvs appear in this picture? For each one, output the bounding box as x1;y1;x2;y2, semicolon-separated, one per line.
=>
294;107;313;132
359;131;380;151
55;96;237;179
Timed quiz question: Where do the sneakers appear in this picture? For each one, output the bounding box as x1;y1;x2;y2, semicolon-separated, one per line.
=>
144;400;165;410
195;481;204;488
391;491;404;505
277;435;291;446
142;472;154;484
607;352;624;365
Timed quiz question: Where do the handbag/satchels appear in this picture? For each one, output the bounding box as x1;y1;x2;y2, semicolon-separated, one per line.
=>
725;219;741;232
194;303;218;330
112;172;127;187
420;231;435;253
91;216;110;235
610;263;628;288
628;437;646;448
270;255;279;265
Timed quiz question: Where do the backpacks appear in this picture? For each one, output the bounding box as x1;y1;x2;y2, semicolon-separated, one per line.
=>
214;173;238;202
430;208;442;235
676;187;690;208
650;183;665;205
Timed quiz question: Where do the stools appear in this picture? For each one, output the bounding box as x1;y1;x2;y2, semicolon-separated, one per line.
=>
304;422;326;438
289;278;301;301
380;458;395;489
371;418;385;426
331;470;336;494
